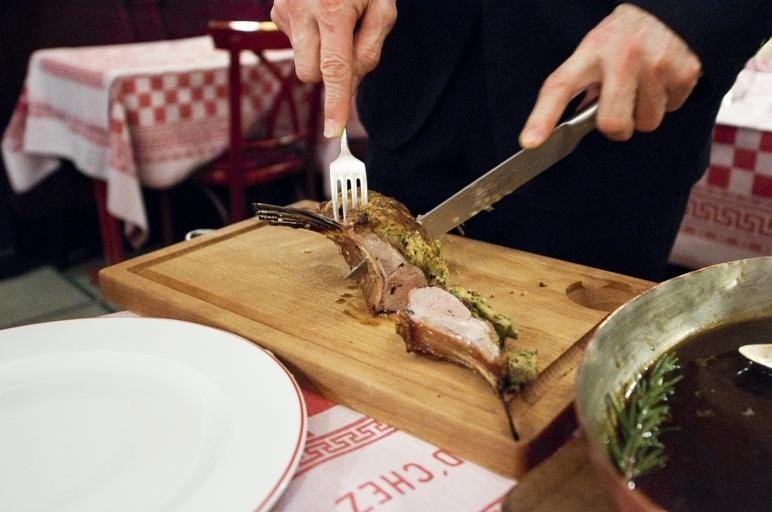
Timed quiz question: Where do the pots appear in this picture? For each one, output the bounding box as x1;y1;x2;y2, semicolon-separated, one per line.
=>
575;256;771;512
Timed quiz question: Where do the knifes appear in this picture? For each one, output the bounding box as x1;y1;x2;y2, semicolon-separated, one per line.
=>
343;102;597;283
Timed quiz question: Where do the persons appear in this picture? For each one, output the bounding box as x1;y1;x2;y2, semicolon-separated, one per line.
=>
269;0;770;281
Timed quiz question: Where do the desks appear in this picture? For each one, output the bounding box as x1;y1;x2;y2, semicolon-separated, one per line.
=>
1;34;317;270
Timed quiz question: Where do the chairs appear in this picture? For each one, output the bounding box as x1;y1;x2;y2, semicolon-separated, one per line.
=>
192;20;323;219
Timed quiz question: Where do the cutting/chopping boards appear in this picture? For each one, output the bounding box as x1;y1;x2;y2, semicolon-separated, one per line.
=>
99;198;660;479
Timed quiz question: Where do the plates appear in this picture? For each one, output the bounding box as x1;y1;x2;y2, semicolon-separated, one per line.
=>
0;317;307;512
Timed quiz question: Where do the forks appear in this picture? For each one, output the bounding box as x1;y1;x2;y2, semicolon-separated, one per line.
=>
328;126;367;224
